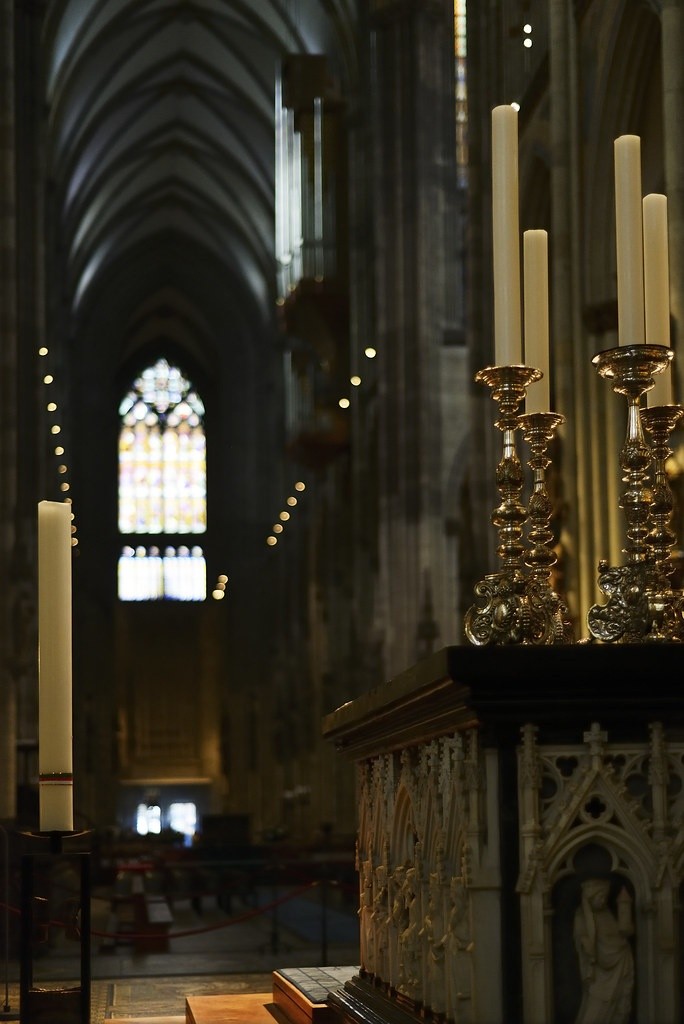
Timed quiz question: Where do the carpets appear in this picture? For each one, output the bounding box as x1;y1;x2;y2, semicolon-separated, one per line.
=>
0;867;363;985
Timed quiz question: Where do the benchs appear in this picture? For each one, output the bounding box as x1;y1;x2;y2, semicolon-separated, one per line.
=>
77;838;363;918
326;968;430;1024
132;876;173;958
271;965;362;1024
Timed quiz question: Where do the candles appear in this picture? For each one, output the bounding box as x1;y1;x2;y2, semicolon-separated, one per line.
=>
36;497;75;835
491;104;524;370
642;193;676;410
613;133;645;349
522;227;549;416
